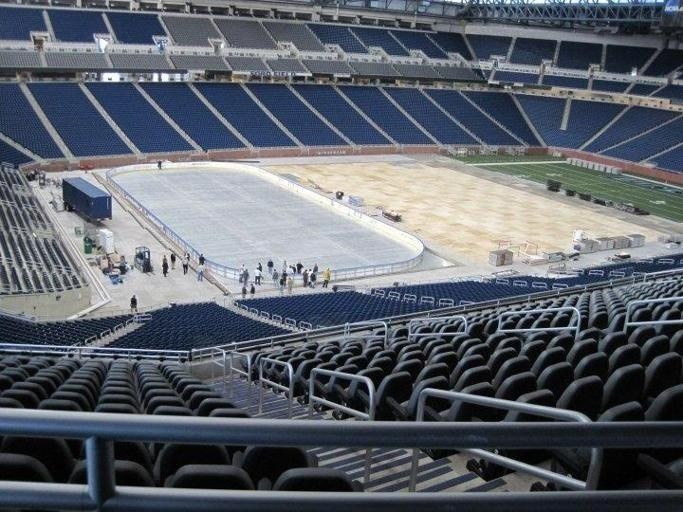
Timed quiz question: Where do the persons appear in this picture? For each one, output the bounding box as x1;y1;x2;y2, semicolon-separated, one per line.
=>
157;160;163;170
197;253;205;265
182;257;187;275
170;253;175;269
184;250;190;261
130;294;137;311
195;262;204;281
162;254;166;266
235;257;331;298
161;260;167;276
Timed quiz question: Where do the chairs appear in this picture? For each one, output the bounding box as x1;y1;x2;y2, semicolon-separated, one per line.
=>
1;256;681;495
0;181;82;255
0;0;682;181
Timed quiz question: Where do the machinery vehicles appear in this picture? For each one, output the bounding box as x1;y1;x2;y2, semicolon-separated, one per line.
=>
129;246;155;277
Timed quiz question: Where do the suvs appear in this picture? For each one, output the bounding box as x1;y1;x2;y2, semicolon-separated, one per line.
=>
607;252;631;263
